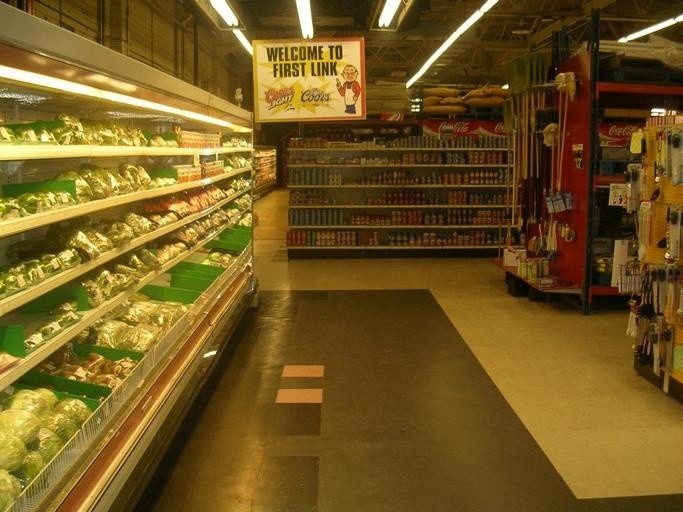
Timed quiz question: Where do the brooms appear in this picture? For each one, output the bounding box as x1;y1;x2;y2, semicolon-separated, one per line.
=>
544;91;573;214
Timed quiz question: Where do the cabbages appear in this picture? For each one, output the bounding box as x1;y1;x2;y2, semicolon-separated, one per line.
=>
0;387;92;512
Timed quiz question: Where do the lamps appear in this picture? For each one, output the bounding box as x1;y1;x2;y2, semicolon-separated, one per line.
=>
195;0;246;32
368;0;414;32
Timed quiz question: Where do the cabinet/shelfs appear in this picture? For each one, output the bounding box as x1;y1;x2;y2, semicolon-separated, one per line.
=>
287;142;520;260
503;47;683;315
0;0;254;512
251;145;279;201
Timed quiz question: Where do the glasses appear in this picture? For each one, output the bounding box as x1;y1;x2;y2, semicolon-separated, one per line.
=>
343;71;356;75
